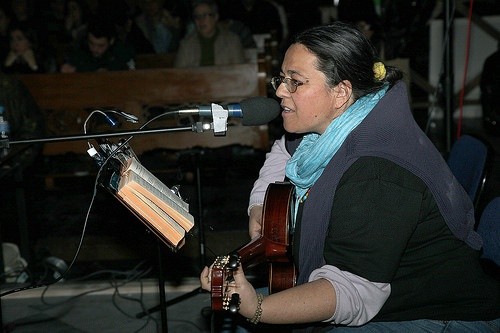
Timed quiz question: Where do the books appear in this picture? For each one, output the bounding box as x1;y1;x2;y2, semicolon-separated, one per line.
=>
116;157;194;247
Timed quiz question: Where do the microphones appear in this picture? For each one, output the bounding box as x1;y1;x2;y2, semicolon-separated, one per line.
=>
83;109;122;150
177;97;280;125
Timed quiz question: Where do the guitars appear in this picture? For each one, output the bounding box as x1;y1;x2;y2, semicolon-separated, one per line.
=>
210;182;298;316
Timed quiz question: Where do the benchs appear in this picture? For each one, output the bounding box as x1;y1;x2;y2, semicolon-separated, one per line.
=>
18;63;269;187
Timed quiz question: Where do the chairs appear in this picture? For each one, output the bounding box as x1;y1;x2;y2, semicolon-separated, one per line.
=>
447;133;500;267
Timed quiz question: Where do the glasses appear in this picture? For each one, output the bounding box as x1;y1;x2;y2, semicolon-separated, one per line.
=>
271;76;305;93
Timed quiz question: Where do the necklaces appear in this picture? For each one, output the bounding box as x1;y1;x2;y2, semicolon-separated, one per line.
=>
299;190;310;202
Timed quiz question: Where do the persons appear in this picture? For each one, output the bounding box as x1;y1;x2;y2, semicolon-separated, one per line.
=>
0;86;57;286
200;23;500;333
0;0;189;74
172;0;246;68
216;0;436;62
479;46;500;198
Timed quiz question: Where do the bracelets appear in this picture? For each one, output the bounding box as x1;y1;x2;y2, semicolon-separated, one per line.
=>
247;292;263;324
